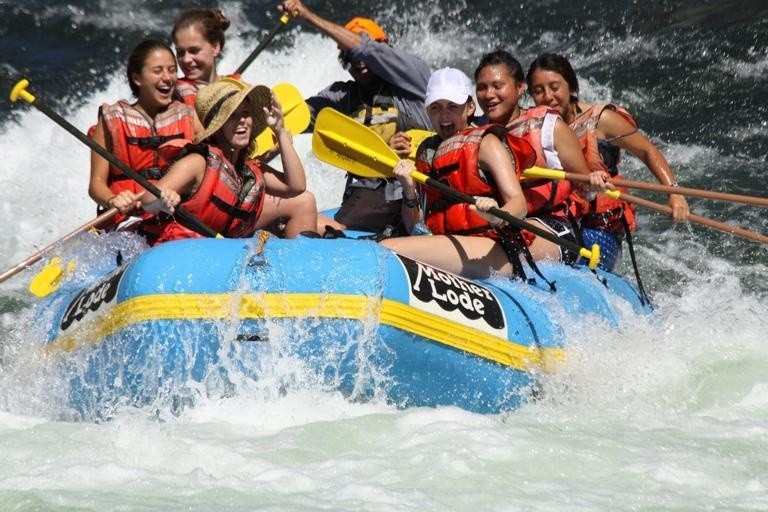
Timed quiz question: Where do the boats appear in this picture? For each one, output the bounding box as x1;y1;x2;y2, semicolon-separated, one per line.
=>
36;205;649;417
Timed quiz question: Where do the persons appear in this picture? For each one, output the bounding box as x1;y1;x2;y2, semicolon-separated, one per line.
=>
276;0;430;235
376;67;525;279
468;53;616;264
88;40;349;235
146;80;307;248
528;56;690;273
167;8;252;137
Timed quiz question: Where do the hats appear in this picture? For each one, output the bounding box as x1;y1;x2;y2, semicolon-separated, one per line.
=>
421;65;478;111
192;78;275;147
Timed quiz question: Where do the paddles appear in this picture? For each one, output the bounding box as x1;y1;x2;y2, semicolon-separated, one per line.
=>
402;129;764;204
312;107;601;271
245;82;312;160
28;226;100;299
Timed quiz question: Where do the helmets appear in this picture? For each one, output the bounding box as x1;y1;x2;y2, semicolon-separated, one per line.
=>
335;16;392;50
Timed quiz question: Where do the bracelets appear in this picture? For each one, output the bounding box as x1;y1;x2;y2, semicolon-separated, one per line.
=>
665;181;681;198
489;216;509;229
